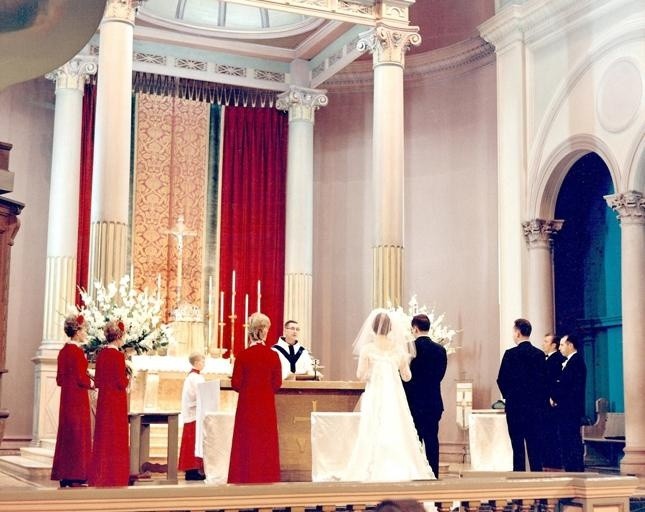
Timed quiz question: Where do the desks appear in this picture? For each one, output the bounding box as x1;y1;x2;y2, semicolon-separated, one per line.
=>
310;413;363;484
469;410;530;472
202;412;236;484
127;410;182;485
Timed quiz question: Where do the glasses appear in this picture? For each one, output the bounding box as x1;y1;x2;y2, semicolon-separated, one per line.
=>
287;327;300;331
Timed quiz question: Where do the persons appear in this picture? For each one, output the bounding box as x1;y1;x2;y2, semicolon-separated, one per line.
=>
400;313;448;479
550;333;588;472
268;319;323;381
226;312;282;483
49;313;93;488
178;350;208;480
543;329;567;472
496;317;548;472
340;305;438;481
88;319;130;486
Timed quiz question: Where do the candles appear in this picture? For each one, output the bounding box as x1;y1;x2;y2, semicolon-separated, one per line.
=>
206;268;262;350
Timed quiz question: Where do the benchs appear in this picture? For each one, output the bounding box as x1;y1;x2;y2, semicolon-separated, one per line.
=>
580;396;626;462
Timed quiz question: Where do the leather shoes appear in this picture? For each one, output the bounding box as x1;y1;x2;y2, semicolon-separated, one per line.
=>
185;473;206;480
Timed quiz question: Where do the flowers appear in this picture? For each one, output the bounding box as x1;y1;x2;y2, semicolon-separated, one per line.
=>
384;293;466;359
54;274;176;364
